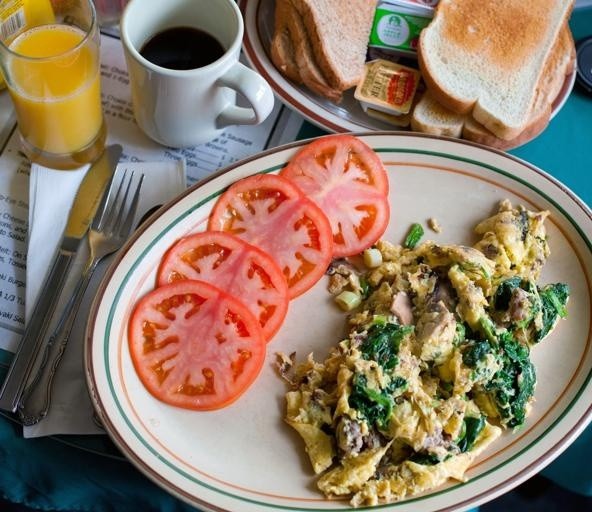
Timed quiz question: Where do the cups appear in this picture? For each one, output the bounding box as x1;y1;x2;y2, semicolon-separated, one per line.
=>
0;0;106;171
118;0;275;148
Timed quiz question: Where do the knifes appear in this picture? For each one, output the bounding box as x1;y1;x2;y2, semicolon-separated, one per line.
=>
1;144;123;411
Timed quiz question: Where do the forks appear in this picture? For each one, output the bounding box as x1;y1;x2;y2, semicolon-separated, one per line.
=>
19;168;147;427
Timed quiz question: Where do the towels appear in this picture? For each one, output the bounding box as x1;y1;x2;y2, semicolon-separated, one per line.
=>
23;156;186;438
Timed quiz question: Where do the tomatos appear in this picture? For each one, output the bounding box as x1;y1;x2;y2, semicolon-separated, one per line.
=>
129;133;389;411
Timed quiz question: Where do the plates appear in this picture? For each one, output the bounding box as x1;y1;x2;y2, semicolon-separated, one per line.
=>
86;133;589;504
